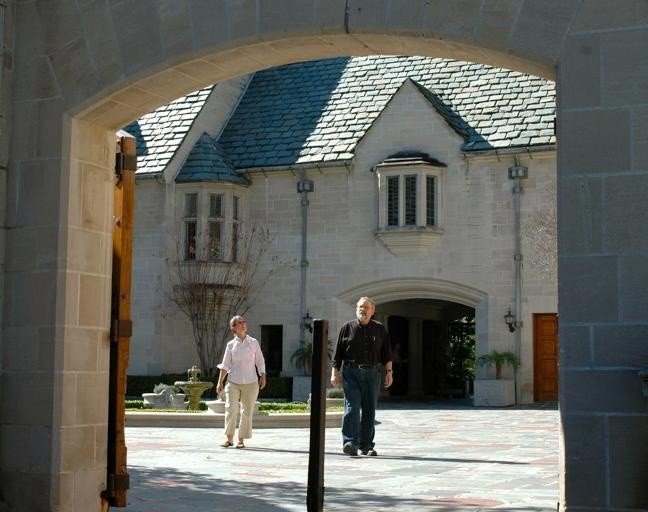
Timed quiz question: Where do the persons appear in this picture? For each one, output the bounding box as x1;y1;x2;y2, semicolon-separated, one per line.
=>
216;316;267;448
330;297;393;456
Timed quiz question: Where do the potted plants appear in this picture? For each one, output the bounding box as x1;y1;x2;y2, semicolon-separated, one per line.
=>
471;351;520;407
291;338;334;403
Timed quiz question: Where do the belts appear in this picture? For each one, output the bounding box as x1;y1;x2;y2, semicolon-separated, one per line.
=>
346;361;382;369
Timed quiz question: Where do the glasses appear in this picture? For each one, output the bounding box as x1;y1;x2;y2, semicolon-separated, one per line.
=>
233;320;246;327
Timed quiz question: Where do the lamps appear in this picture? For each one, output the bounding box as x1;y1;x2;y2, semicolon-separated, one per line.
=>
303;308;313;333
503;303;515;332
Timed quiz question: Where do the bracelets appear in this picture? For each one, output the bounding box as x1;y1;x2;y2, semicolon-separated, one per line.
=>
387;369;393;374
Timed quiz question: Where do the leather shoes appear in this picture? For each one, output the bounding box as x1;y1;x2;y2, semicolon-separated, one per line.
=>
361;449;377;456
344;444;358;455
219;441;244;448
